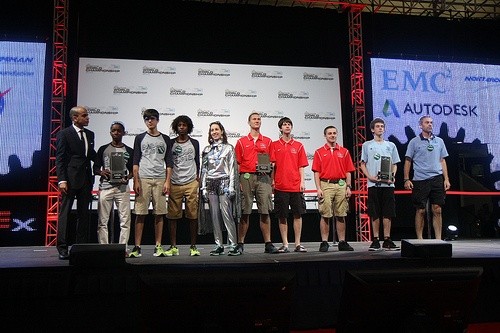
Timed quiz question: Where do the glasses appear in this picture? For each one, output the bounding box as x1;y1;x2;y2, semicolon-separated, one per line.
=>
145;117;158;120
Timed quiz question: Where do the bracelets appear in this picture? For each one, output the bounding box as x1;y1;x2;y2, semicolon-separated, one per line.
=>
347;184;352;188
404;178;409;184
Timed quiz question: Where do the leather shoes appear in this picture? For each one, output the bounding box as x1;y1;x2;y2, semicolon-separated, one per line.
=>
59;251;67;259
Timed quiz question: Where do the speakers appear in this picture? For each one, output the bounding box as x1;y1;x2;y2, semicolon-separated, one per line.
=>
69;244;127;269
400;239;453;258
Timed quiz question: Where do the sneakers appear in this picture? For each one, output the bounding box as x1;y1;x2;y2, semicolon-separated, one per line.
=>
129;246;142;258
338;242;354;251
368;239;381;251
210;247;224;256
153;244;165;257
162;246;180;257
264;242;279;254
318;242;329;252
236;243;244;253
228;245;240;255
381;239;401;251
189;246;200;256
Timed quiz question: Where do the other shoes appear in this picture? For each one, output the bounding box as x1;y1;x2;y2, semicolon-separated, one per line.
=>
278;246;290;253
294;245;307;252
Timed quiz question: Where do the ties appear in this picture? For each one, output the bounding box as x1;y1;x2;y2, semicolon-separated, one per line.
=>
79;130;86;155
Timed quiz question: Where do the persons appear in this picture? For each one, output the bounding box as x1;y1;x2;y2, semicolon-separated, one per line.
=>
269;117;309;252
162;115;200;257
200;122;236;256
359;118;401;251
55;106;104;259
129;109;174;257
234;113;279;255
311;126;356;253
403;115;450;239
93;121;134;258
0;261;500;333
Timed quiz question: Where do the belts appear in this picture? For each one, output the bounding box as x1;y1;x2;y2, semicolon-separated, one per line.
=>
321;178;340;183
